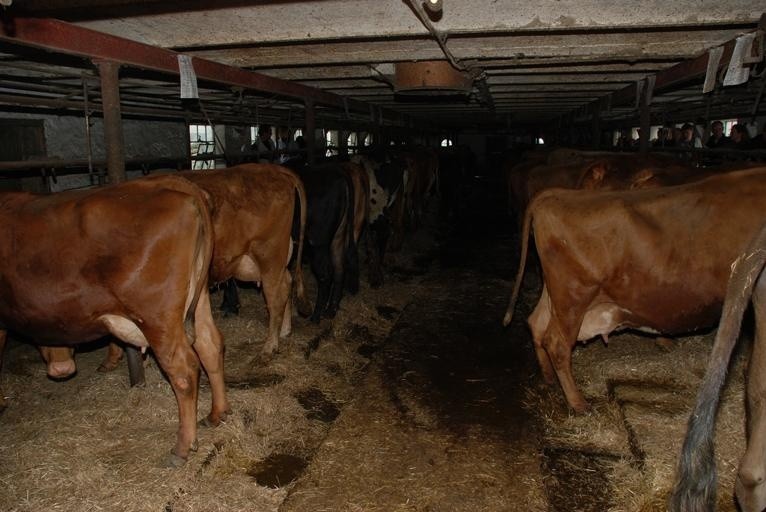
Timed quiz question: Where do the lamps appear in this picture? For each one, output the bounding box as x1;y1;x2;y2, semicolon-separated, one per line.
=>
393;62;473;96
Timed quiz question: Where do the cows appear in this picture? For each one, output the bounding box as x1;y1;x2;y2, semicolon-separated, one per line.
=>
502;146;765;512
0;140;446;469
